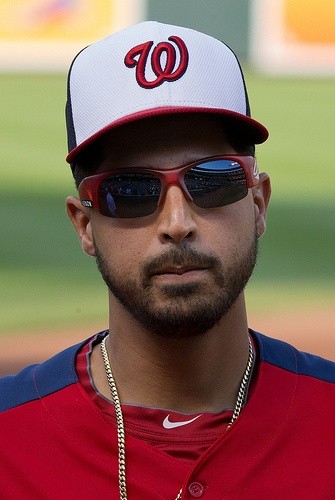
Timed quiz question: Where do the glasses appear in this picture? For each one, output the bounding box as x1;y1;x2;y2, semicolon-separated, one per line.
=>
77;156;258;218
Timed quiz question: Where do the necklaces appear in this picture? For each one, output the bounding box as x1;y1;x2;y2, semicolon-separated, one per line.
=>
99;332;257;500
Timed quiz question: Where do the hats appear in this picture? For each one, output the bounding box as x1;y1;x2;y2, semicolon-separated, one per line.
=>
65;19;268;160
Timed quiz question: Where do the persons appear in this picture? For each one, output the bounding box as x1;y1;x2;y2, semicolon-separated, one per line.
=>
0;18;335;500
